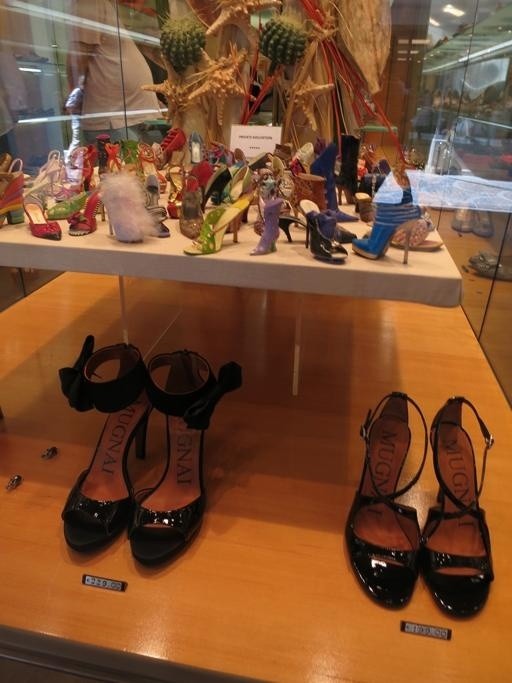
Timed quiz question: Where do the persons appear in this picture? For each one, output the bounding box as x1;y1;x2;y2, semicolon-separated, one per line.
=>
1;50;27;172
67;0;164;151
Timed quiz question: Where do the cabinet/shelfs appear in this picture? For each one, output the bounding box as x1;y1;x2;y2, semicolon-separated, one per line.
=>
0;214;512;683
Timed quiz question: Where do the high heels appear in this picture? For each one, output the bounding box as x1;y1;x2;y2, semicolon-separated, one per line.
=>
346;391;494;619
0;130;445;265
62;342;216;564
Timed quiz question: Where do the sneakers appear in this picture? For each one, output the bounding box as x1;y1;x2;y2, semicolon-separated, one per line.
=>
432;85;512;126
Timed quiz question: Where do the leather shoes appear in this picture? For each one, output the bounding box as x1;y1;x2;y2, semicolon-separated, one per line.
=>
418;166;512;281
